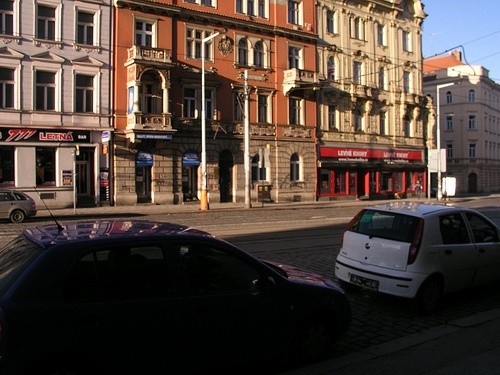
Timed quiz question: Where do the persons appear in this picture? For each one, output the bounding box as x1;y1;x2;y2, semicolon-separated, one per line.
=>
414;180;423;200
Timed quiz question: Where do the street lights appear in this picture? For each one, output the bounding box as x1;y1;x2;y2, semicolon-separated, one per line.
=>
436;81;455;202
199;31;220;210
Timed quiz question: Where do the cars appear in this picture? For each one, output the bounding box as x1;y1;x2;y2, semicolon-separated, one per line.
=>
0;188;38;224
0;220;353;375
334;199;500;313
